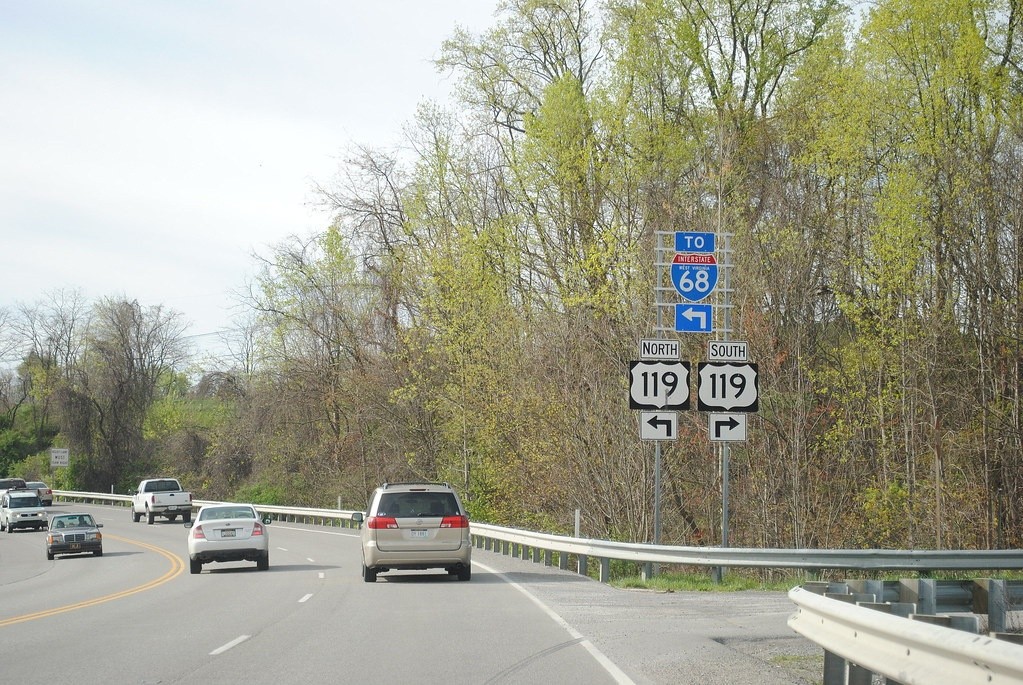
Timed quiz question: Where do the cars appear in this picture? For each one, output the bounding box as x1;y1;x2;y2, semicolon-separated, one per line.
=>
23;482;55;506
43;513;104;560
184;503;271;575
0;490;49;534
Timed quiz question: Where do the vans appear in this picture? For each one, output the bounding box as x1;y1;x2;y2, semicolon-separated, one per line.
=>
0;477;27;506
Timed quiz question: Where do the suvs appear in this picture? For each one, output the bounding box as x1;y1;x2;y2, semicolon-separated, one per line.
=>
352;481;472;581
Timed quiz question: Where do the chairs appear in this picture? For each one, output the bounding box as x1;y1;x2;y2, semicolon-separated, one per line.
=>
430;502;443;516
57;520;65;528
388;504;401;515
79;517;88;526
207;511;217;519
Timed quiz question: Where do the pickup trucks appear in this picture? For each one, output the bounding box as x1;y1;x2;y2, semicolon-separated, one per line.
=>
131;478;193;526
